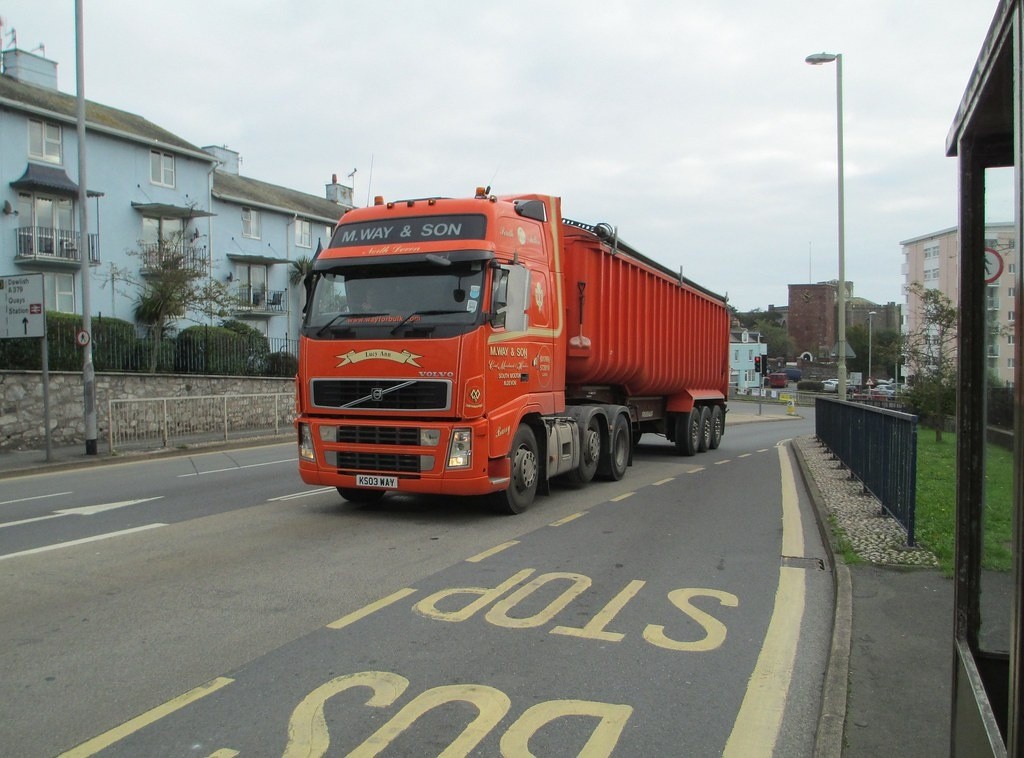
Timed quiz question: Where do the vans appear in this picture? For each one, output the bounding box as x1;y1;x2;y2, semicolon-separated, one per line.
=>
771;373;788;388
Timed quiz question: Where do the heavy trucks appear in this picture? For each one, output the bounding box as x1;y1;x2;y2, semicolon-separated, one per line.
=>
292;185;731;516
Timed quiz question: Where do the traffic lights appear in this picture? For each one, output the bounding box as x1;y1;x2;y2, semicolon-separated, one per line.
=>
755;356;760;372
762;354;767;377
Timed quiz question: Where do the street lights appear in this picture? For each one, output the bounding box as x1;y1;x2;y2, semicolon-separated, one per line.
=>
805;52;847;404
867;311;877;400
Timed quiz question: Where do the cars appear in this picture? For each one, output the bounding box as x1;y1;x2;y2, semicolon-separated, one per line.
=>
821;379;857;394
863;379;911;400
